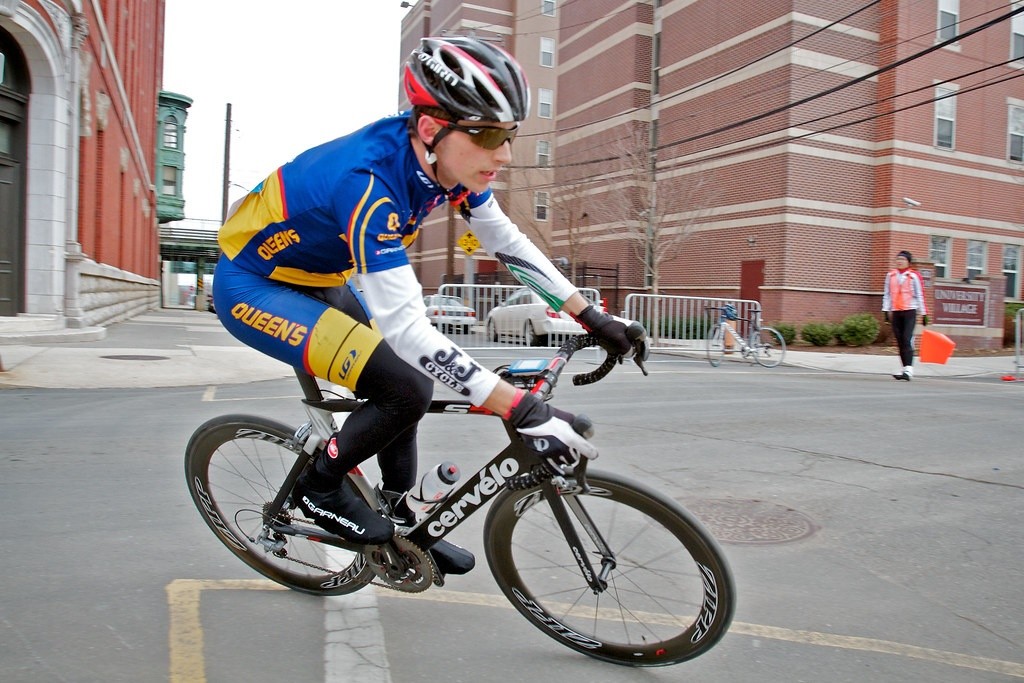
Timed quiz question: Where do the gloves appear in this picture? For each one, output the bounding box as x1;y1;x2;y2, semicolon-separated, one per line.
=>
508;391;599;478
575;305;649;363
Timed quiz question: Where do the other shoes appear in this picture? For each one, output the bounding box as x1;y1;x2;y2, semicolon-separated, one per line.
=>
893;366;915;381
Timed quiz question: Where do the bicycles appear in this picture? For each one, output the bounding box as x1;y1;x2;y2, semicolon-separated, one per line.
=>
704;303;785;369
186;326;738;670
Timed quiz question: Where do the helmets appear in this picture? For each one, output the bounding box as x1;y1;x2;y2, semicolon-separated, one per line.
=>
406;37;529;122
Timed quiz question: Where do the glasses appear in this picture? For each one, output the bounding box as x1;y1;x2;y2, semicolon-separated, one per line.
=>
434;118;520;150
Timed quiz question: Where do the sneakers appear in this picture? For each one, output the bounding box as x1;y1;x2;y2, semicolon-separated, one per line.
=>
431;536;476;574
293;472;394;543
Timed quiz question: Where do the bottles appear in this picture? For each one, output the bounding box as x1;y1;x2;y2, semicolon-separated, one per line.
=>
405;461;460;514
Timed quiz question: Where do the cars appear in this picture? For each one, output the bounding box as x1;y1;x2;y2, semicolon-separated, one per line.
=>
423;295;476;334
485;288;608;347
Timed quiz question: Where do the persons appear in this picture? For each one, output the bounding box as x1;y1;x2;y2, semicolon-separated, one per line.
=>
882;251;928;382
212;34;652;576
